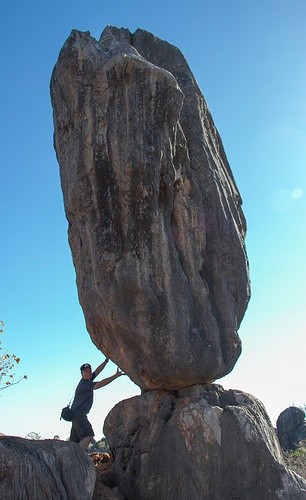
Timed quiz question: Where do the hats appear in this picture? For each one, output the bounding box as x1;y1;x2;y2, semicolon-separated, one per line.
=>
80;363;91;371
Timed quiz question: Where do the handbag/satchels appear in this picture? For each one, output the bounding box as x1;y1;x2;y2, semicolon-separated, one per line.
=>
60;406;75;421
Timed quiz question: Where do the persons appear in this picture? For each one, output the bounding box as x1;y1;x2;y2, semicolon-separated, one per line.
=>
69;358;128;449
